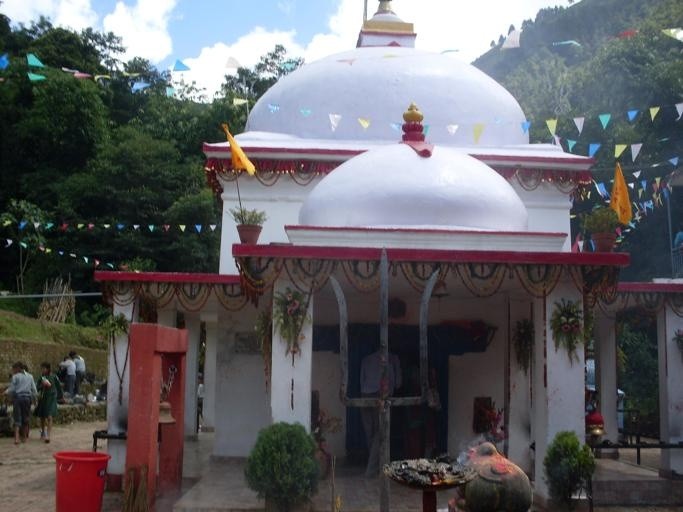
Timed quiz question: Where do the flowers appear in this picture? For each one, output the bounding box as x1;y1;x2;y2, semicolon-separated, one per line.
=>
472;396;508;447
546;294;588;366
309;408;345;460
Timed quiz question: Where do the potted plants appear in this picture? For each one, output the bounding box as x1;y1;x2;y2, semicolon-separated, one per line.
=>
583;203;622;252
227;206;267;244
270;287;310;356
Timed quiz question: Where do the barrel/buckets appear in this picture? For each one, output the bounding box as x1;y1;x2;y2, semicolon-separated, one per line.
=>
53;450;112;512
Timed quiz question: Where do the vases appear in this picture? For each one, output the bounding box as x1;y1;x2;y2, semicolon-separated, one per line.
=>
311;442;330;480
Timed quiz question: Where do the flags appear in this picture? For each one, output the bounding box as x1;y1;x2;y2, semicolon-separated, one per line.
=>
225;129;254;176
610;163;631;225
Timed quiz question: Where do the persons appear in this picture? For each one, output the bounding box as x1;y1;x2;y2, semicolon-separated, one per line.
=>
3;351;85;443
